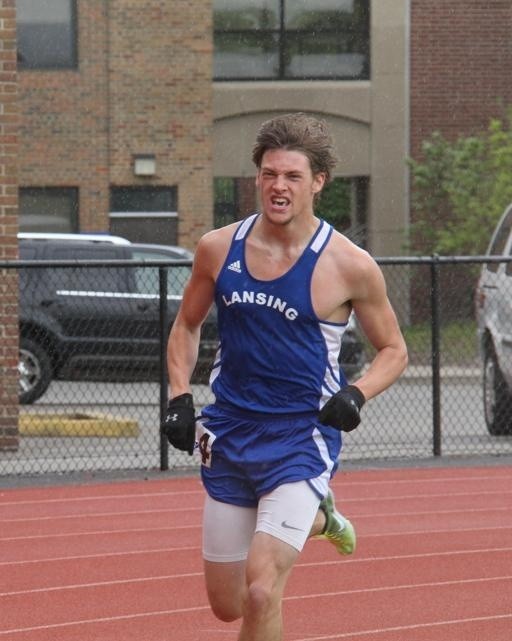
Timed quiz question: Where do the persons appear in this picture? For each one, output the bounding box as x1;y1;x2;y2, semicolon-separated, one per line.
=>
162;111;408;641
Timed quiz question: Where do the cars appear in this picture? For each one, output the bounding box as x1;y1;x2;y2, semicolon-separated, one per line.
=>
474;202;512;436
17;231;368;406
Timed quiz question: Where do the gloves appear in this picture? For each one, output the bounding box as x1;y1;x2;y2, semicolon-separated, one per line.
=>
319;385;367;434
160;393;199;457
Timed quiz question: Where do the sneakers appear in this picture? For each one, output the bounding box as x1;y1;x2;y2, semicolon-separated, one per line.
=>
317;484;357;557
